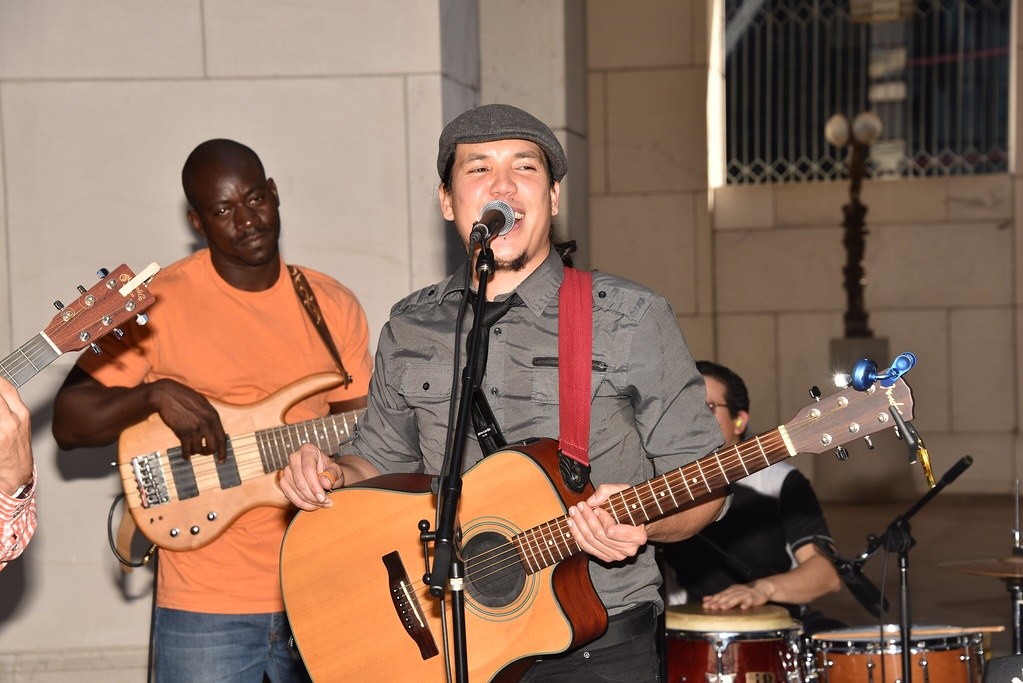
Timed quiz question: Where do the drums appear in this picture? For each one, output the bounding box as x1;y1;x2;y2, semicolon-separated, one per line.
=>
810;624;985;682
663;602;819;683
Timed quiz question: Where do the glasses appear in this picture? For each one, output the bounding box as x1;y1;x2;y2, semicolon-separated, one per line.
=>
704;403;731;413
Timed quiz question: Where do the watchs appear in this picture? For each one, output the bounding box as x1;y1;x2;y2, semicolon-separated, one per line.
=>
10;476;33;500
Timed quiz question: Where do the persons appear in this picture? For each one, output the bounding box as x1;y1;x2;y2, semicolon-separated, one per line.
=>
278;103;738;683
0;376;39;582
51;138;377;683
653;361;849;633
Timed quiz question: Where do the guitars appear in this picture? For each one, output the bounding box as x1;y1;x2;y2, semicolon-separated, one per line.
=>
278;352;937;683
0;262;163;391
114;371;369;552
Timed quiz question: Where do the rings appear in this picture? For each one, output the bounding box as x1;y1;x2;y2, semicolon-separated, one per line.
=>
318;473;336;489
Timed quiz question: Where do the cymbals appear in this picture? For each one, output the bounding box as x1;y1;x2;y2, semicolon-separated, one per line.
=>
937;554;1023;577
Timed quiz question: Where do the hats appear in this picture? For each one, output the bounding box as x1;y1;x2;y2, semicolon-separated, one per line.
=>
437;103;569;184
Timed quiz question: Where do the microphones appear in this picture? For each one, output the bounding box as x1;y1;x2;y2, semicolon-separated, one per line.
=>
815;538;889;621
469;201;516;243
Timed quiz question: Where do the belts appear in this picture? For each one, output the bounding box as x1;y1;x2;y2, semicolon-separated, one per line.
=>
579;601;657;653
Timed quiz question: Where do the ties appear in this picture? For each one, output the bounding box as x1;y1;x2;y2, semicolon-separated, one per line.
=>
465;292;508;386
